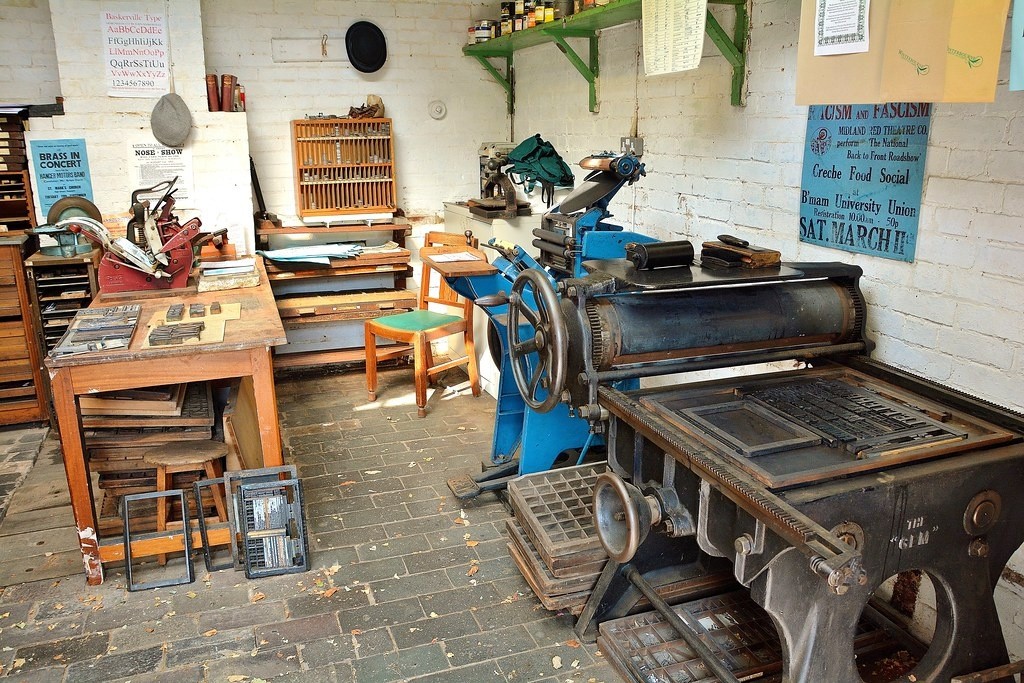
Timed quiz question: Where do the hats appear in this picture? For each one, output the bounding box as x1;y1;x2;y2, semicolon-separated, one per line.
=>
151;93;190;147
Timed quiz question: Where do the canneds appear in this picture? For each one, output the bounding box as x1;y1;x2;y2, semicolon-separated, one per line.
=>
469;0;554;45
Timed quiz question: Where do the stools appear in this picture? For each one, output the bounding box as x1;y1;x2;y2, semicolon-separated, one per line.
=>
142;440;232;566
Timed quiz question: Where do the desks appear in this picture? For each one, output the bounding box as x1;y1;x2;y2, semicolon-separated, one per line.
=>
45;254;288;586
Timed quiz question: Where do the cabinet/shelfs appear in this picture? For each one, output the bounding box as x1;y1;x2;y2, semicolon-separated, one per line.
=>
253;209;419;388
444;201;542;401
289;117;397;217
0;170;104;439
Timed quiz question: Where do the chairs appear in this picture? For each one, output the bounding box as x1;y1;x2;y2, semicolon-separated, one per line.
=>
366;232;481;419
